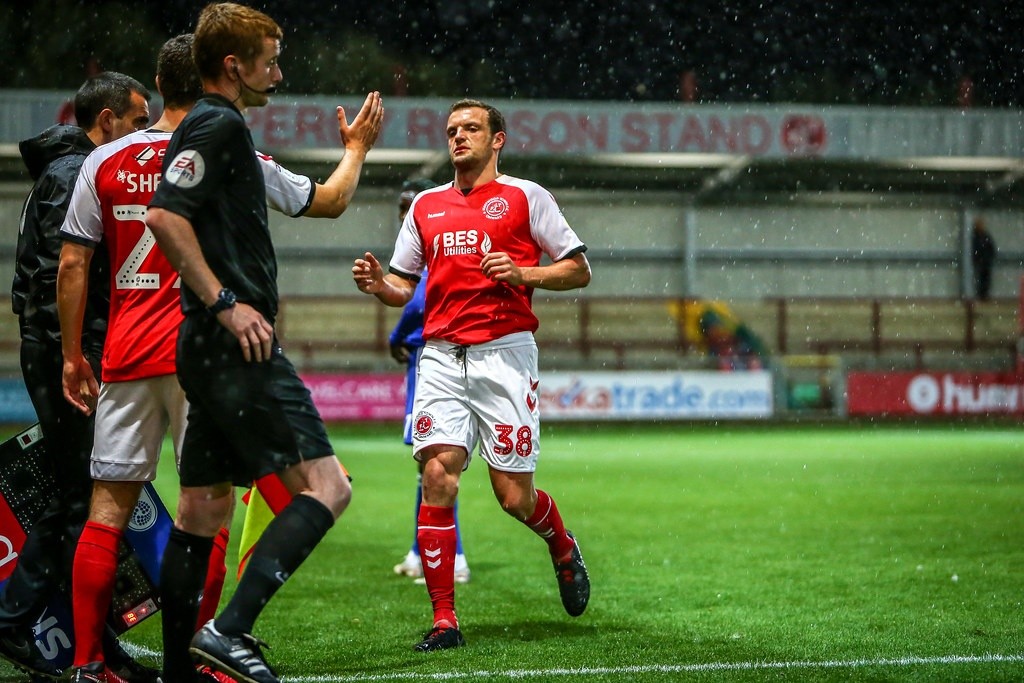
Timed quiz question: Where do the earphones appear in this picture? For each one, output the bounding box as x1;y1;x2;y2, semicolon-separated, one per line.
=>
230;60;238;73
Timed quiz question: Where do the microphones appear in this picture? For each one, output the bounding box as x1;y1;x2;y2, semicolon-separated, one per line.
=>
237;74;277;94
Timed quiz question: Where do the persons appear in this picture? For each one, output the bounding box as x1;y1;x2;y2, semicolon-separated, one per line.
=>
385;178;473;584
0;68;160;683
972;216;998;303
348;99;595;652
137;0;353;683
57;29;384;683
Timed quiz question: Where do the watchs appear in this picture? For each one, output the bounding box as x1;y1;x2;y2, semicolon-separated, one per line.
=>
205;287;238;317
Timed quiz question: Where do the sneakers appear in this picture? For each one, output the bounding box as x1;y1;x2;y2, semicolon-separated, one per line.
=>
416;627;465;650
71;661;128;683
394;550;424;577
414;553;470;584
188;618;279;683
552;530;590;617
